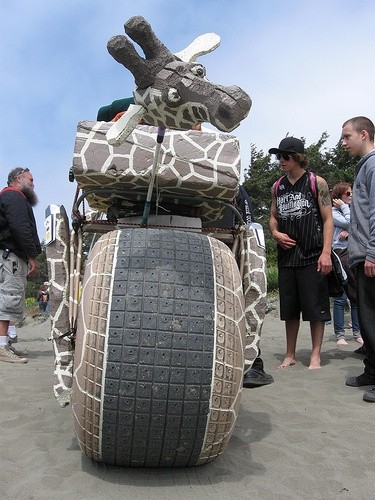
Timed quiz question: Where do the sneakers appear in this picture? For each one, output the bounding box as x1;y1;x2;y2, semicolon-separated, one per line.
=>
0;344;28;363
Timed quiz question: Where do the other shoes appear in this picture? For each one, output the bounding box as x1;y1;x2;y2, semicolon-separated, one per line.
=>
345;375;375;387
363;387;375;402
6;333;18;343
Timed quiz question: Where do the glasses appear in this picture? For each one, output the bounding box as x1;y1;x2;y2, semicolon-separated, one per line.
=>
343;191;353;197
277;154;294;161
20;168;30;175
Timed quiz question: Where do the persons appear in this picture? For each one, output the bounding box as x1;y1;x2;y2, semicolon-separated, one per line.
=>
0;168;42;363
36;285;49;312
337;116;375;404
268;137;334;370
330;182;365;347
235;185;275;387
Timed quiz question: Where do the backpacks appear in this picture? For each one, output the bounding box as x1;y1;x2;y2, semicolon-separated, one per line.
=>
39;291;47;302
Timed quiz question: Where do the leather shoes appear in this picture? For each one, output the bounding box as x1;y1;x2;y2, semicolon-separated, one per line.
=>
243;368;274;385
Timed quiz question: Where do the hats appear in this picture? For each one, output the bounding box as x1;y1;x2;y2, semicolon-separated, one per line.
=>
268;136;304;154
44;282;49;285
